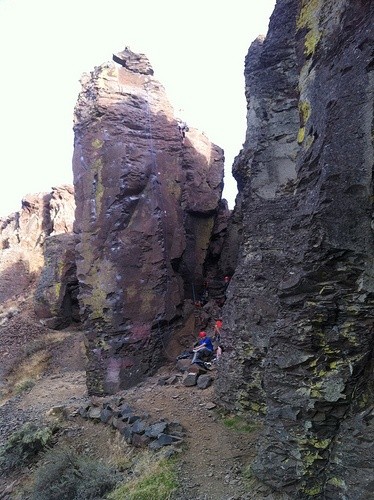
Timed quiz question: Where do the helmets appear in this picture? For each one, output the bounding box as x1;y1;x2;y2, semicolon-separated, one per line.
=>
198;332;206;338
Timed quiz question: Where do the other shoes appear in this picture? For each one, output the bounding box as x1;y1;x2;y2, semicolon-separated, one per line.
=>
189;349;195;354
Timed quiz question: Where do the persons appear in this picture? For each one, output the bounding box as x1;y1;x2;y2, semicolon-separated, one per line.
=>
213;321;224;362
191;331;214;364
224;277;229;284
